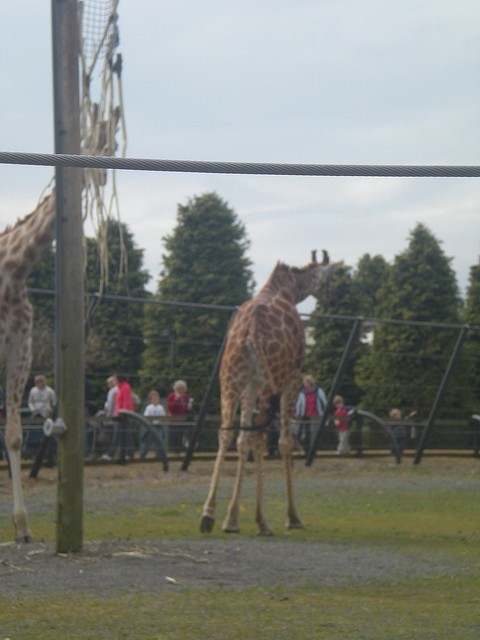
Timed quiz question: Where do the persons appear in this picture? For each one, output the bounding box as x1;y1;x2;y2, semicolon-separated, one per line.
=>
104;375;118;424
295;374;329;455
166;380;189;456
28;374;56;417
101;372;134;461
333;395;352;455
386;408;417;456
132;392;140;412
140;390;167;459
265;411;281;459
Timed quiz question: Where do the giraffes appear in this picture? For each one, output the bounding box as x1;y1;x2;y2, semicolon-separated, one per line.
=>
1;103;121;545
199;250;344;535
